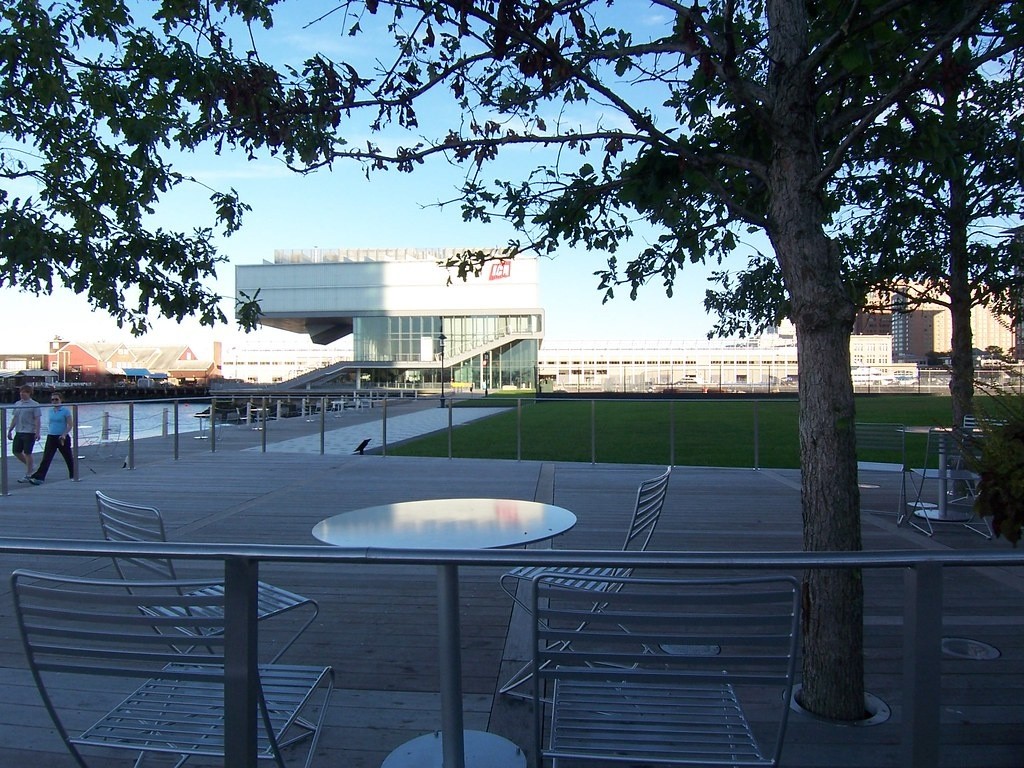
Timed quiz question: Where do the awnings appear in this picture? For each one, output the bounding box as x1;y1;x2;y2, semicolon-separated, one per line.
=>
149;373;168;379
105;367;127;383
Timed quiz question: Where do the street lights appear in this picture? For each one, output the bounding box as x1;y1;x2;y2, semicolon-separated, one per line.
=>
484;352;493;397
436;334;447;409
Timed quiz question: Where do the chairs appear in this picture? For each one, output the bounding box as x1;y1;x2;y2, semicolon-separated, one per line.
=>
10;406;1007;768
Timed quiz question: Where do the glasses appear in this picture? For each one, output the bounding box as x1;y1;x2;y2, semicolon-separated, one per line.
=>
51;398;60;401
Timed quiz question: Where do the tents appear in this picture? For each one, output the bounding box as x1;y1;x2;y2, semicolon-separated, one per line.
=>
124;368;151;384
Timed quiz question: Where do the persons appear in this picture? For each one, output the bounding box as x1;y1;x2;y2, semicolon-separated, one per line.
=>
29;391;74;485
8;385;43;483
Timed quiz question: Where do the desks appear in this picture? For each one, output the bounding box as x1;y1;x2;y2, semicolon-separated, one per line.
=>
249;408;269;428
895;426;991;522
333;401;347;414
193;415;210;438
312;496;578;768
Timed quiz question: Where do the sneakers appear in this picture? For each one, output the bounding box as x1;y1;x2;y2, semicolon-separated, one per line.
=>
71;479;81;481
17;475;31;482
30;478;43;484
